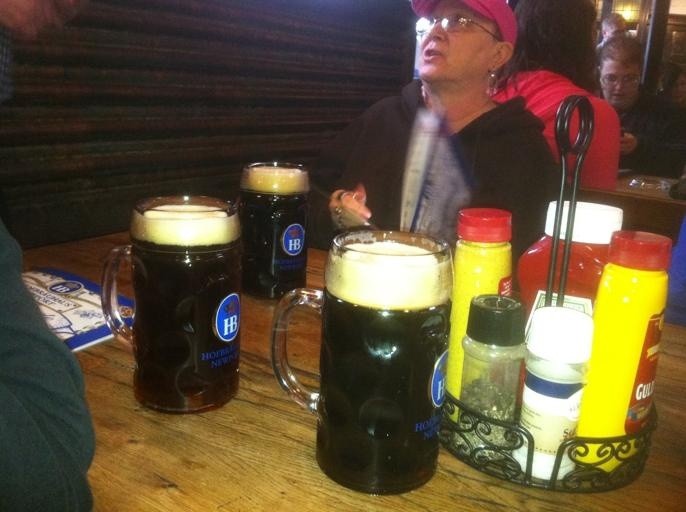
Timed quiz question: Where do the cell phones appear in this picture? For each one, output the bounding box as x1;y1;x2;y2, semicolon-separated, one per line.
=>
619;126;626;136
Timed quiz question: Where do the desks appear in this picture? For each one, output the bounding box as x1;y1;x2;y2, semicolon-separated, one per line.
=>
572;172;686;246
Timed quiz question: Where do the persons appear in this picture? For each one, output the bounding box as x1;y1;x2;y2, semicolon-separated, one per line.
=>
488;0;622;192
0;0;95;512
305;1;555;269
585;10;686;181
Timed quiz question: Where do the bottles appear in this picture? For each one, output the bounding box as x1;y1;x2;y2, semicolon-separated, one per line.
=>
446;201;672;480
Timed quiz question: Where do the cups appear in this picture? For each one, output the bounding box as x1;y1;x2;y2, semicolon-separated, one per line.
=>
243;161;308;299
100;195;244;412
272;231;453;495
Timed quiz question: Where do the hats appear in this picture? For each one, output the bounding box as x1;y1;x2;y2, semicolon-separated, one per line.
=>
412;0;517;44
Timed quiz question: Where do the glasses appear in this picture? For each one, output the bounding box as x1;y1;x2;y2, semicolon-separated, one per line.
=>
600;73;641;89
415;13;500;42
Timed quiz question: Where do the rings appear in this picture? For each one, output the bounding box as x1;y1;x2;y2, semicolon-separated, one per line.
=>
334;190;350;231
